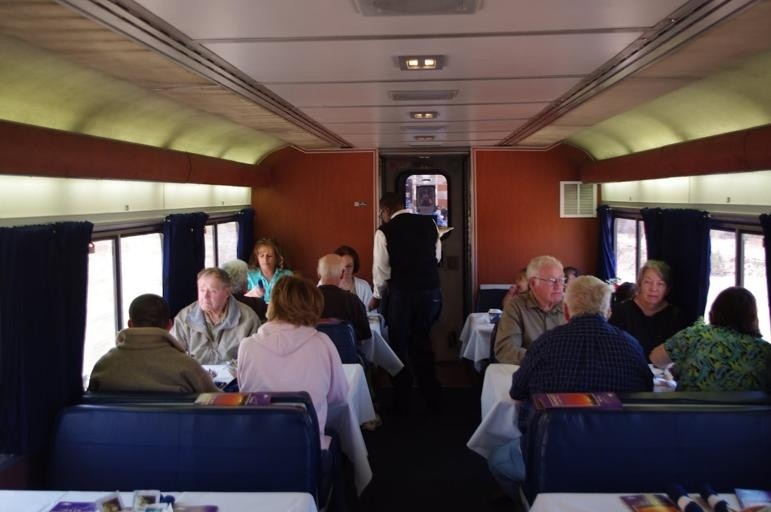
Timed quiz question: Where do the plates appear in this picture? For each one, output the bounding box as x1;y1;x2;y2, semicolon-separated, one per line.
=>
488;309;502;314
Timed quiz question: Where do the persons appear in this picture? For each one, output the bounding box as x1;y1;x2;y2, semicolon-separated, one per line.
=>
649;286;771;392
316;244;374;310
406;191;449;226
315;254;372;346
493;255;685;364
218;258;269;327
370;192;444;420
89;292;222;393
487;274;655;502
235;276;350;472
169;267;260;384
245;238;294;304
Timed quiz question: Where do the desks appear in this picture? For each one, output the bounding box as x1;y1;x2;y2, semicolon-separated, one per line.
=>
357;312;405;377
459;312;504;373
466;363;678;484
529;488;771;512
200;363;376;498
0;489;319;512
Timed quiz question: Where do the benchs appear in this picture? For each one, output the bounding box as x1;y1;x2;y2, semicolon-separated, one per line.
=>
519;391;771;512
476;284;516;312
480;318;500;372
44;393;334;512
315;319;357;363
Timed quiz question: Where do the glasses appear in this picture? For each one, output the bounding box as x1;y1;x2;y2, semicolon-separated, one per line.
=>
532;276;569;289
